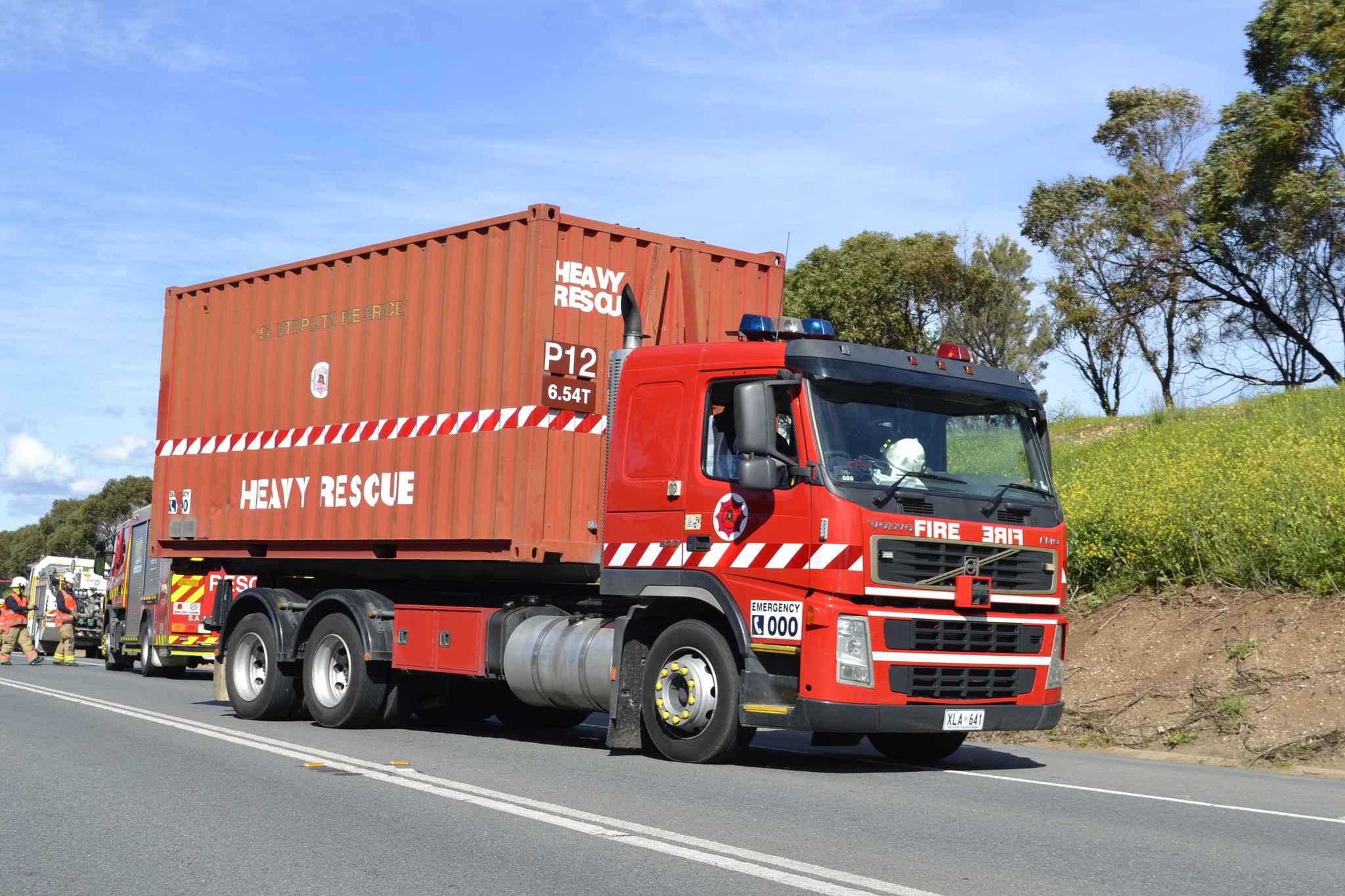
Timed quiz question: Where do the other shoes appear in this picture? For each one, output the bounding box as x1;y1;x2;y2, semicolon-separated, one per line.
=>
54;662;67;666
2;661;12;665
67;663;78;667
30;657;44;665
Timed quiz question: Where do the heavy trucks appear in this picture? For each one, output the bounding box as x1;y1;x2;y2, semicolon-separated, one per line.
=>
151;201;1069;767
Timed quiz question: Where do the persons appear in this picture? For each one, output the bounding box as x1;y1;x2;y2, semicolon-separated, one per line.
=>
51;572;81;666
0;576;44;665
733;390;789;485
0;599;5;645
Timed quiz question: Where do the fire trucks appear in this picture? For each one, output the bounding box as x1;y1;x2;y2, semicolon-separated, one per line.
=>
94;503;239;677
21;554;110;657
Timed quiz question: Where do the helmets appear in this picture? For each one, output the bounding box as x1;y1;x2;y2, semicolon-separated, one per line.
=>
880;438;924;471
61;572;75;584
0;599;5;604
9;576;27;587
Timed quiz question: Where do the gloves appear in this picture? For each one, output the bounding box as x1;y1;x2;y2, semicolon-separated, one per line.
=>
27;605;34;609
70;611;79;620
